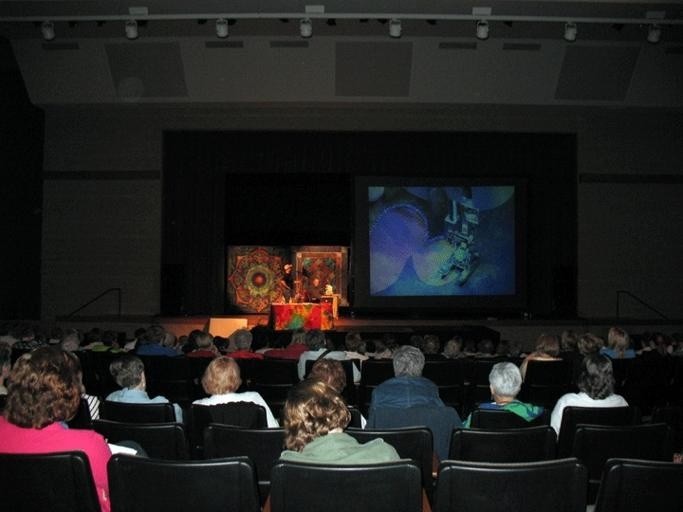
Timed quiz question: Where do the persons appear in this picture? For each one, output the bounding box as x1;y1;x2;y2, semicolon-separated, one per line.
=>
304;277;321;303
264;377;433;511
279;264;296;303
0;344;115;511
0;324;681;435
